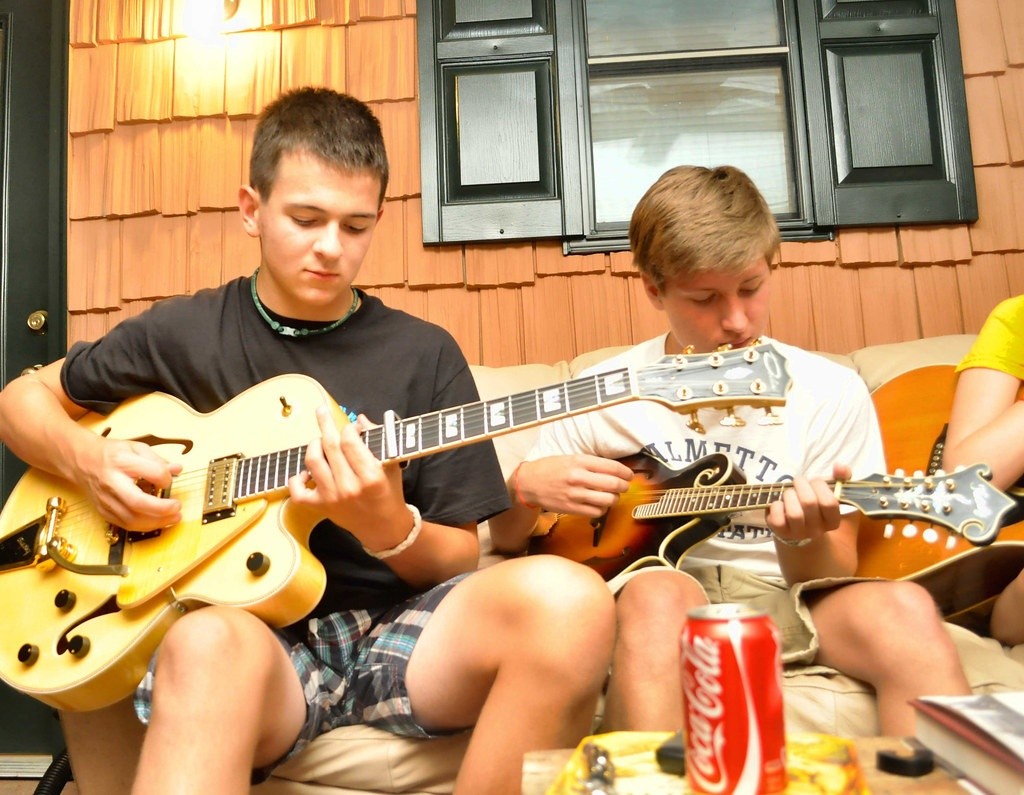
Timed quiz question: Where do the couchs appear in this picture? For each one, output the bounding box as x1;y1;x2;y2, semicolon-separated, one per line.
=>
62;334;1024;795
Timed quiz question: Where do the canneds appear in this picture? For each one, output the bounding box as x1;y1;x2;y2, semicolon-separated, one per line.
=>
678;602;789;795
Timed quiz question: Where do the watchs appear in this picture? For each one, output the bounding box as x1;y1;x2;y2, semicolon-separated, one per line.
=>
772;533;811;547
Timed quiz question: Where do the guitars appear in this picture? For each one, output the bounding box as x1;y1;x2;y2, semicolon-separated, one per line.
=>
528;452;1018;602
0;339;793;713
853;364;1024;621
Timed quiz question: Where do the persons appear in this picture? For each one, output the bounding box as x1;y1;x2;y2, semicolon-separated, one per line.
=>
488;163;973;738
942;294;1024;648
0;89;619;795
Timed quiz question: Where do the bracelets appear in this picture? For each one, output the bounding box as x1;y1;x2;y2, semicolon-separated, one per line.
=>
364;503;422;558
